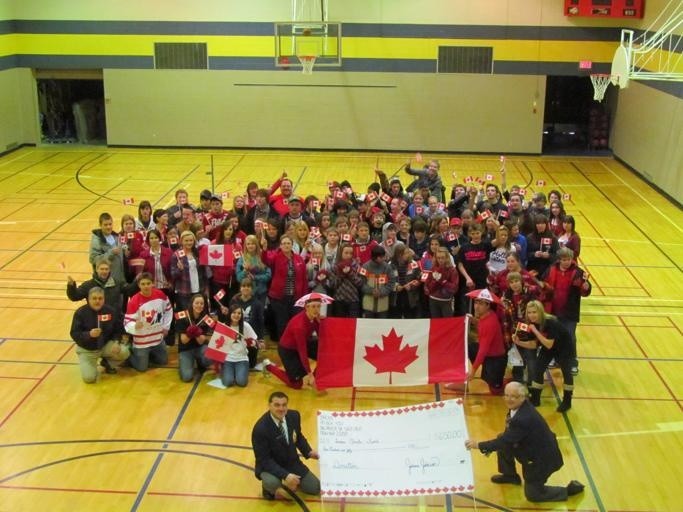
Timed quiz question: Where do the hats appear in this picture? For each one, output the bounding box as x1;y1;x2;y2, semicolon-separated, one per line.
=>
288;195;304;205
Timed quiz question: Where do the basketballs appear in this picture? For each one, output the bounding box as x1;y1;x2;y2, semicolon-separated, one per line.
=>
588;108;608;147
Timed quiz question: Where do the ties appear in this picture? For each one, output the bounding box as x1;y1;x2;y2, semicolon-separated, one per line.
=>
278;421;287;443
506;408;512;429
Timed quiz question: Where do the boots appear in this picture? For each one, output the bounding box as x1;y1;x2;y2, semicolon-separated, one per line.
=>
529;381;545;407
557;384;573;412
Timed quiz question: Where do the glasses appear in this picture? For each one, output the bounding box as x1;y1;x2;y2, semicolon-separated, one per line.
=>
503;393;519;401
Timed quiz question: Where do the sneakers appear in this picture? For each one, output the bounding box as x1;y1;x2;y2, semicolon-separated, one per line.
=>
255;359;270;371
101;358;116;373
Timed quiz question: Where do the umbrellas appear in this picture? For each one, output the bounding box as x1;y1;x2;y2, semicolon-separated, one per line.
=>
464;287;503;306
292;290;335;308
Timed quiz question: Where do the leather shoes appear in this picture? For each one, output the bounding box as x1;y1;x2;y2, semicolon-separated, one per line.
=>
262;487;275;500
568;480;584;493
491;474;521;485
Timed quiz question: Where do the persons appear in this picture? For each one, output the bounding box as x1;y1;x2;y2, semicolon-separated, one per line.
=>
252;393;320;500
66;259;122;345
265;219;282;250
391;246;421;318
320;182;331;213
218;304;258;387
221;214;248;250
143;230;174;304
456;224;496;315
465;381;586;503
507;186;565;236
175;293;213;382
70;286;131;384
194;190;212;233
263;298;321;390
330;245;366;318
468;298;510;396
243;188;283;234
290;220;324;289
266;169;305;218
260;234;309;339
254;218;266;248
494;254;534;303
359;245;396;318
467;177;507;243
170;230;203;313
308;213;331;247
135;200;155;242
331;180;354;245
414;161;467;258
557;215;581;265
118;215;142;274
236;235;273;351
424;247;458;317
153;209;169;249
528;213;559;281
124;272;173;372
243;182;258;209
207;221;235;312
228;195;248;235
89;213;123;286
166;190;192;233
303;192;321;231
418;238;455;316
354;159;414;265
319;229;341;270
503;271;540;384
506;223;528;270
166;206;204;249
538;247;592;376
280;198;316;234
488;226;516;312
511;300;575;413
195;196;232;231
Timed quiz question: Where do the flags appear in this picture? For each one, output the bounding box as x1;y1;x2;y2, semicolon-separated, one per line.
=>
125;232;136;240
563;194;571;200
204;314;215;328
123;198;134;205
419;272;430;283
221;192;229;199
535;180;545;187
540;237;552;245
311;200;320;207
175;310;189;320
204;321;239;363
516;322;529;332
376;274;387;285
141;309;152;318
174;309;189;320
259;223;268;230
358;267;368;277
232;250;242;259
141;310;153;318
203;314;215;327
198;244;233;267
168;237;177;245
315;317;470;391
